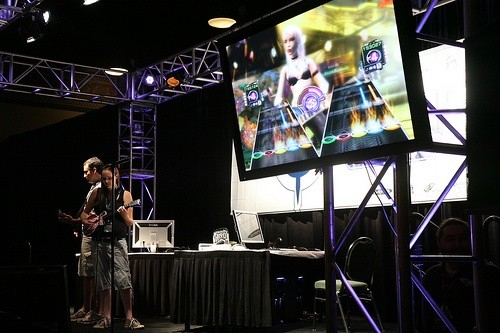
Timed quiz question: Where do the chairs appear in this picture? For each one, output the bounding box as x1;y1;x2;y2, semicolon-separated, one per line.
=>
313;236;384;333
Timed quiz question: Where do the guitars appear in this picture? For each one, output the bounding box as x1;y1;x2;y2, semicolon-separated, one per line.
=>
56;208;80;240
82;198;142;237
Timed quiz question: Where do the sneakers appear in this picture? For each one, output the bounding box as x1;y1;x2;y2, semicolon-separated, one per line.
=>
125;317;144;328
71;308;88;321
78;310;101;324
94;317;111;328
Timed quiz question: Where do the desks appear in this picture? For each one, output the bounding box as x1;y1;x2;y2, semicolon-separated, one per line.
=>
73;249;326;333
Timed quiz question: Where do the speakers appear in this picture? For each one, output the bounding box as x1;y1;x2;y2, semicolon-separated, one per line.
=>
0;265;72;333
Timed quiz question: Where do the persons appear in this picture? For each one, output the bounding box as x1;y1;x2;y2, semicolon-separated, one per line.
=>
420;218;500;333
80;164;145;329
63;157;105;322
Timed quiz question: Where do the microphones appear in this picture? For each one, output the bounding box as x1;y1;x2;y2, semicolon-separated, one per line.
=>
103;155;134;168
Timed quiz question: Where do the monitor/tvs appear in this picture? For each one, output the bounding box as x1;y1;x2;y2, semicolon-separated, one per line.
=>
218;0;432;182
132;219;174;252
233;210;265;249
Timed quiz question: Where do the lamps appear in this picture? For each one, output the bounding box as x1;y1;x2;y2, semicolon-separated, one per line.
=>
18;12;48;45
167;72;181;87
207;0;240;29
